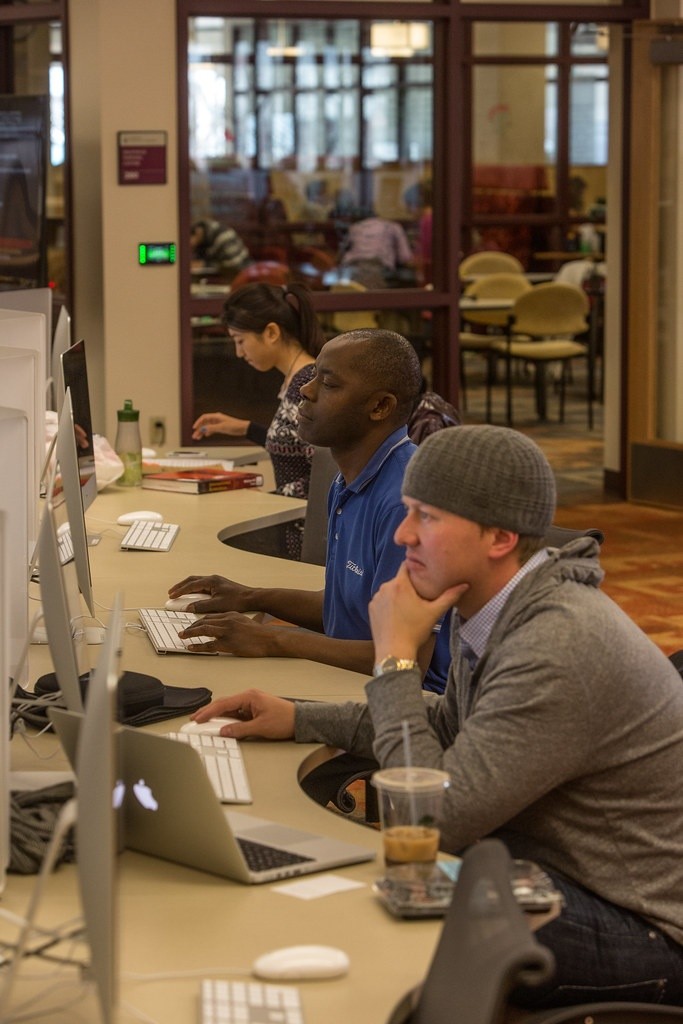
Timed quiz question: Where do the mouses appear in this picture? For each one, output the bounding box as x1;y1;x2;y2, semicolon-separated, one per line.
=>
165;594;212;612
180;716;244;736
253;945;348;979
117;511;163;525
140;447;156;458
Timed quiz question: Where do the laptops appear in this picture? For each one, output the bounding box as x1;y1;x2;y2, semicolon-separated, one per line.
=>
43;708;375;883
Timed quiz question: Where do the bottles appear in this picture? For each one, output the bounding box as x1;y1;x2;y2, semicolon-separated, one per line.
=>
112;400;143;488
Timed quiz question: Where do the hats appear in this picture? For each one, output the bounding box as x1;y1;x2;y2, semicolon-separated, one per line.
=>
400;425;556;536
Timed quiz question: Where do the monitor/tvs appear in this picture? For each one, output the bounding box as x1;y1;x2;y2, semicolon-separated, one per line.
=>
0;302;127;1024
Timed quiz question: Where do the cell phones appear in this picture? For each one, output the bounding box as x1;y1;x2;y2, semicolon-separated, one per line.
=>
166;450;209;459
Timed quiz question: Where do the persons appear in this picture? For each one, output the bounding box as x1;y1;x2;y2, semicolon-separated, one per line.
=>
470;175;601;273
168;328;452;694
186;424;683;1024
408;377;464;447
189;180;433;293
191;282;327;563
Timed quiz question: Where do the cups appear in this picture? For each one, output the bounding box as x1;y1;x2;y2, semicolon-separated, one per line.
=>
370;767;452;881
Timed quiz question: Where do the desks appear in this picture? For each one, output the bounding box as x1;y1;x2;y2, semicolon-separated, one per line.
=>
0;447;561;1024
534;252;605;259
457;298;516;414
458;273;555;287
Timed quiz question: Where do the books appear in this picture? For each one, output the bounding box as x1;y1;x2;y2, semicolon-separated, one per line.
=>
373;862;557;922
142;470;263;495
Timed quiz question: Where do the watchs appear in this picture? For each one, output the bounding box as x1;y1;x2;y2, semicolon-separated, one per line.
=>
373;655;414;677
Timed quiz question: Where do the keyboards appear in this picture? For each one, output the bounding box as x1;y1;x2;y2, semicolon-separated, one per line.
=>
164;732;253;806
199;978;303;1024
138;608;221;656
120;519;180;551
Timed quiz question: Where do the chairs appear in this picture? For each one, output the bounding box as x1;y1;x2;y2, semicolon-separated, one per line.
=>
401;838;557;1023
297;440;341;568
184;245;606;432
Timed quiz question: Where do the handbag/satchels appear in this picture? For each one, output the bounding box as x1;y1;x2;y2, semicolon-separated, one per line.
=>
34;671;168;718
